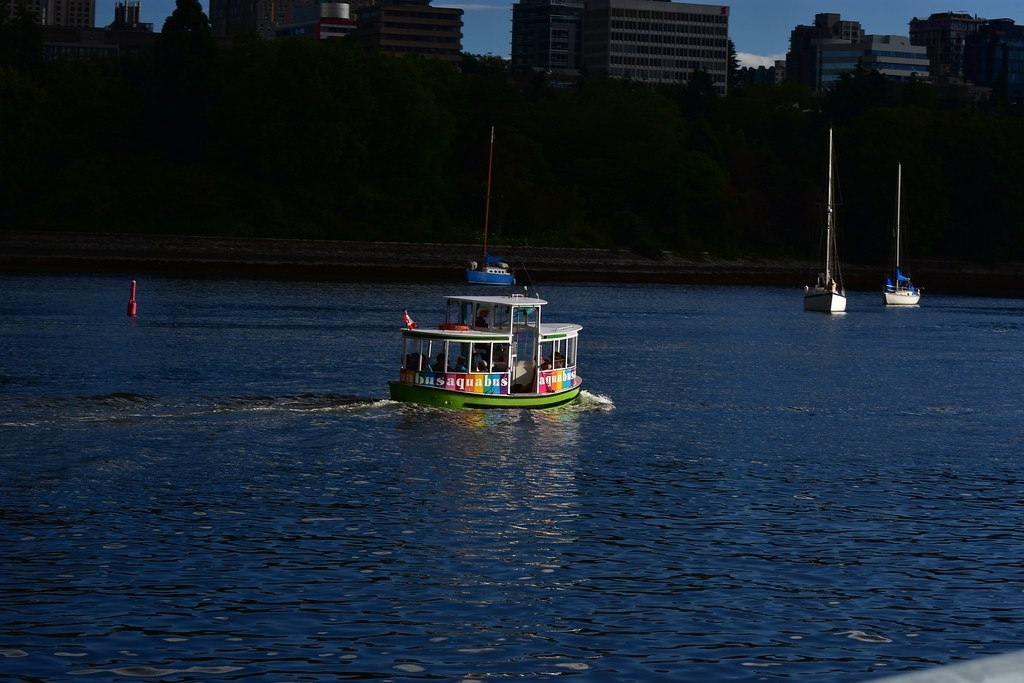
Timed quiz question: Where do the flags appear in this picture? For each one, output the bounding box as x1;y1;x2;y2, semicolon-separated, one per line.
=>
403;310;417;331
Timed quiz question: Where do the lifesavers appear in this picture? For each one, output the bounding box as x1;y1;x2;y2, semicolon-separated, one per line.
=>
438;323;471;332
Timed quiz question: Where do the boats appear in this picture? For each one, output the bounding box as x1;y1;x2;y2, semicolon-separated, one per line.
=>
386;294;584;410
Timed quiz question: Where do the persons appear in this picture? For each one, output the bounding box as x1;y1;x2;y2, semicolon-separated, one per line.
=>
533;355;552;370
475;305;489;328
402;352;489;373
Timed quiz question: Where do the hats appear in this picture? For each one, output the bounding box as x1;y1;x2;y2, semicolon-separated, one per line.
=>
480;306;489;311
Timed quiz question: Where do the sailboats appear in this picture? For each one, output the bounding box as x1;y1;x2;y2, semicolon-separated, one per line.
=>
803;127;848;315
884;162;921;307
466;122;516;285
